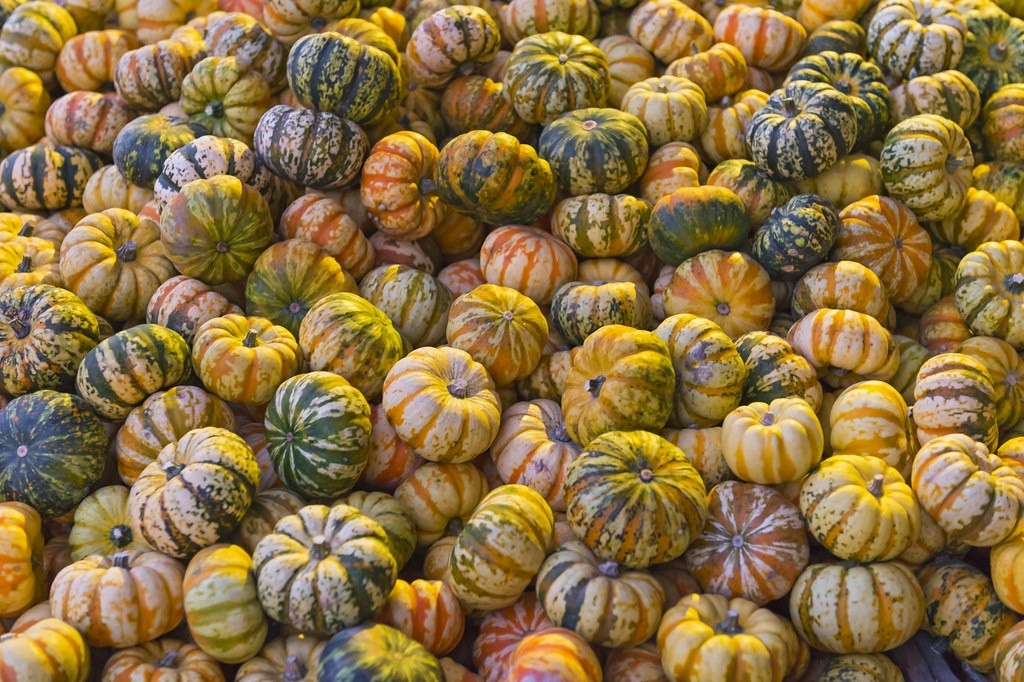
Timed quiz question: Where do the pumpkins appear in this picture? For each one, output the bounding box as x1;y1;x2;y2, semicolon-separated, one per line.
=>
0;0;1024;682
